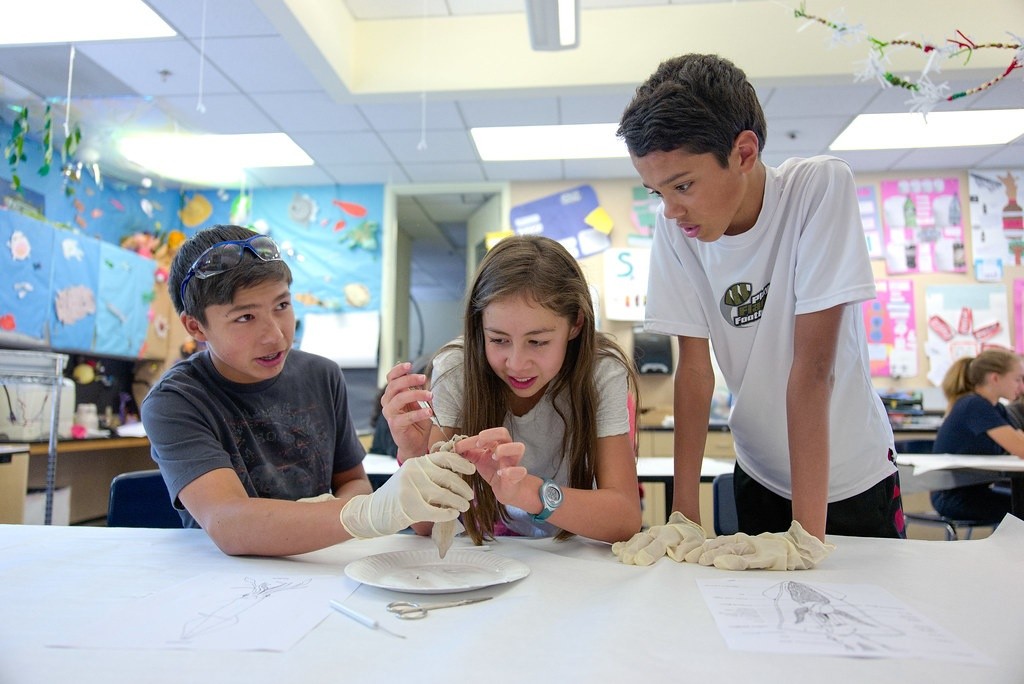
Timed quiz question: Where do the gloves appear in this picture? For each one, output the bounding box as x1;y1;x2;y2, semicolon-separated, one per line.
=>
611;511;836;572
340;451;476;540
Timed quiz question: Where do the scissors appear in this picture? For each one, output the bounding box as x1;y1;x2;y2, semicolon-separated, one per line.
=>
386;596;494;620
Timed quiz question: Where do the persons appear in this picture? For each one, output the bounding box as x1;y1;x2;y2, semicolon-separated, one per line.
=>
140;223;476;557
378;234;643;545
927;347;1024;524
610;53;908;571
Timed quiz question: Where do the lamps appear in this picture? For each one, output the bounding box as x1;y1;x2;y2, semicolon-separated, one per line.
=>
525;0;580;53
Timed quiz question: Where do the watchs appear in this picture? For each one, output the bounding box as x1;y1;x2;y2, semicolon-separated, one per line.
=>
525;477;563;524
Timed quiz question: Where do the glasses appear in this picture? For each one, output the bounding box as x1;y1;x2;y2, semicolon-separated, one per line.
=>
180;234;283;315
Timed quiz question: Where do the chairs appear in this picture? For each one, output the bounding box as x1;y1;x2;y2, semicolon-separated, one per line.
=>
902;511;998;539
106;471;182;528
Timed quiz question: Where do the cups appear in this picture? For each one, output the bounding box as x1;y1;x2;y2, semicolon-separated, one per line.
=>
76;403;98;431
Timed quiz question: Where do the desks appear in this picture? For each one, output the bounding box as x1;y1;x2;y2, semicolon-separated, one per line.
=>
896;452;1024;520
635;454;734;526
1;438;151;524
0;524;1024;684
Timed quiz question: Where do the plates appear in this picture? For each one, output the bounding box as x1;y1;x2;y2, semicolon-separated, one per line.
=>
342;548;531;595
